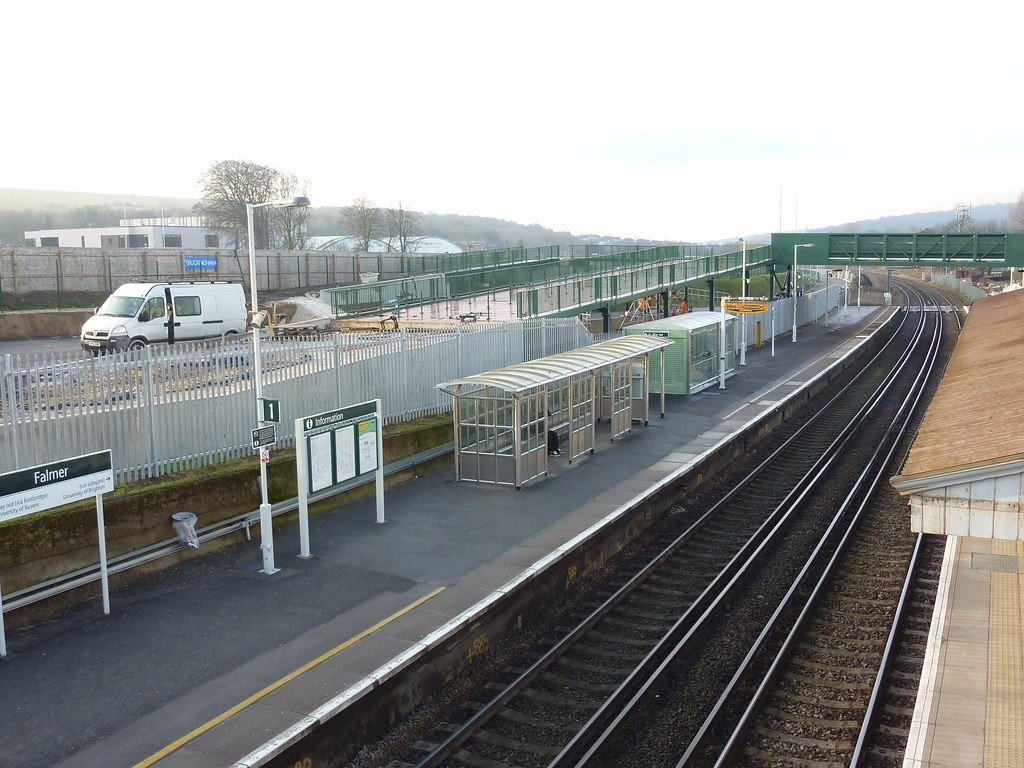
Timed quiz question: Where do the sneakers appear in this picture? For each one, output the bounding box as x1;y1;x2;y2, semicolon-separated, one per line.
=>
556;448;564;453
550;450;560;456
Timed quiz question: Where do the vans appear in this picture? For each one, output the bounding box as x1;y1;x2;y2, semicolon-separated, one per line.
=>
80;274;248;363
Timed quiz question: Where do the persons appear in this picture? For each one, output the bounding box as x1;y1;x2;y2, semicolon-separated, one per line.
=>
531;410;564;457
637;298;650;316
143;303;154;320
680;298;687;313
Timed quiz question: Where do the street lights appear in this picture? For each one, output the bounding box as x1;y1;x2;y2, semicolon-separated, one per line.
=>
790;243;815;343
737;236;748;366
245;196;313;575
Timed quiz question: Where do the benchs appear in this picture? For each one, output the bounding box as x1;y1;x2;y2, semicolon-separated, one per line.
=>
493;411;590;454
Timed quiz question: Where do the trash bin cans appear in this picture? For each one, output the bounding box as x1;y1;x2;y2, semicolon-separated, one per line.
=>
171;511;199;549
